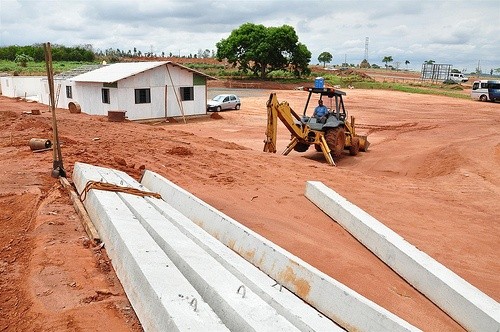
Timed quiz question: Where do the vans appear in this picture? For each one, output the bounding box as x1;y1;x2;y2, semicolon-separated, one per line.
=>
471;79;500;102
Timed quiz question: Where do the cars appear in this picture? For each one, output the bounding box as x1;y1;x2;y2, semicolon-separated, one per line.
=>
207;94;241;112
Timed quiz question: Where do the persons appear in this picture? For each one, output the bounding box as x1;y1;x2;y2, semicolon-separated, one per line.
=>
312;99;328;124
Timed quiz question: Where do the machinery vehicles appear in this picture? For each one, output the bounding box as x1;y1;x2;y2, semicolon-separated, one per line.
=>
263;86;371;168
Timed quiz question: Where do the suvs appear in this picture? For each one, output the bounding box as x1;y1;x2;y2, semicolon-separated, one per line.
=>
450;73;469;82
